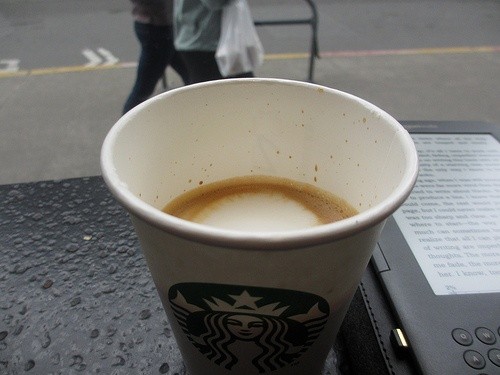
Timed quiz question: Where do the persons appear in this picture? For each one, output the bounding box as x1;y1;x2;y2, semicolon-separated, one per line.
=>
121;1;191;117
172;0;253;83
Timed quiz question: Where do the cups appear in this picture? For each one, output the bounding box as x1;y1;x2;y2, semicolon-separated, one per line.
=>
101;77;420;375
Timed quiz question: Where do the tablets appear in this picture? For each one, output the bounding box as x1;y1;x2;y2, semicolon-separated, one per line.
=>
371;119;499;375
